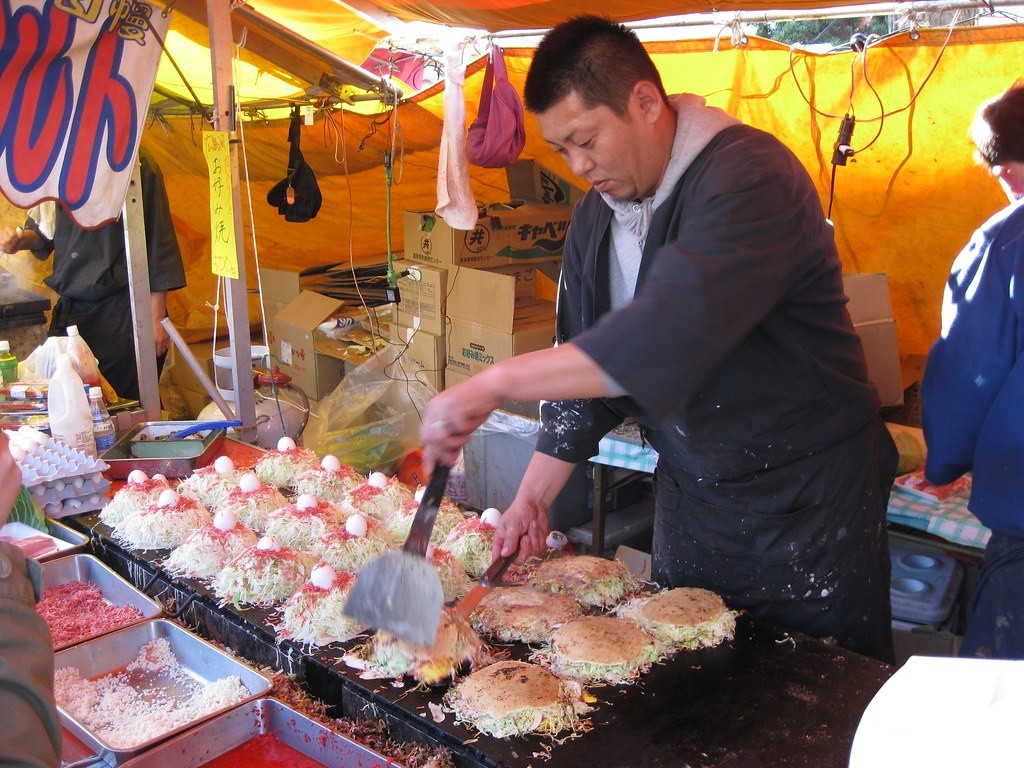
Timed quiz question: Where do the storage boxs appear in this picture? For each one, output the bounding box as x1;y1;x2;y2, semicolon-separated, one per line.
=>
842;268;905;405
262;159;590;419
890;544;965;665
460;416;632;532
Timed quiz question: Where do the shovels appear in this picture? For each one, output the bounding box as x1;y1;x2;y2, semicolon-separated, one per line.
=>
342;425;535;681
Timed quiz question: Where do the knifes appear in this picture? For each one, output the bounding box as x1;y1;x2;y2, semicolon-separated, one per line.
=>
455;547;519;619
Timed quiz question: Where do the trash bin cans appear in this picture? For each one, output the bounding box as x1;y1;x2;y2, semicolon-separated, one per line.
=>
461;397;593;532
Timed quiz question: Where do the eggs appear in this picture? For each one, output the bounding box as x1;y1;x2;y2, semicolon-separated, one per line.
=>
128;437;503;589
4;425;104;514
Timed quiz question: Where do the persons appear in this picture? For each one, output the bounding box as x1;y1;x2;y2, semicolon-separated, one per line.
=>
0;145;188;410
418;14;900;663
0;430;63;768
921;84;1024;660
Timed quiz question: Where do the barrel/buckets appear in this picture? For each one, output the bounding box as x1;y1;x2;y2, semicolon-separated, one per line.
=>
48;354;98;460
66;324;107;406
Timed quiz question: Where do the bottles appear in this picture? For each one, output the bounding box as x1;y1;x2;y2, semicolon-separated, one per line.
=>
0;341;19;384
83;384;92;406
88;386;116;456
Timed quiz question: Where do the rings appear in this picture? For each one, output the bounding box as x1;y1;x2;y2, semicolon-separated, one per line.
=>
430;419;446;430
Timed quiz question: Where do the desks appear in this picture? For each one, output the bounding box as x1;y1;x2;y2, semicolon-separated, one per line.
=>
587;425;994;564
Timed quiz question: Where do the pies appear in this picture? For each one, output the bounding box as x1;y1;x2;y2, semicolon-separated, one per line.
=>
373;552;724;713
